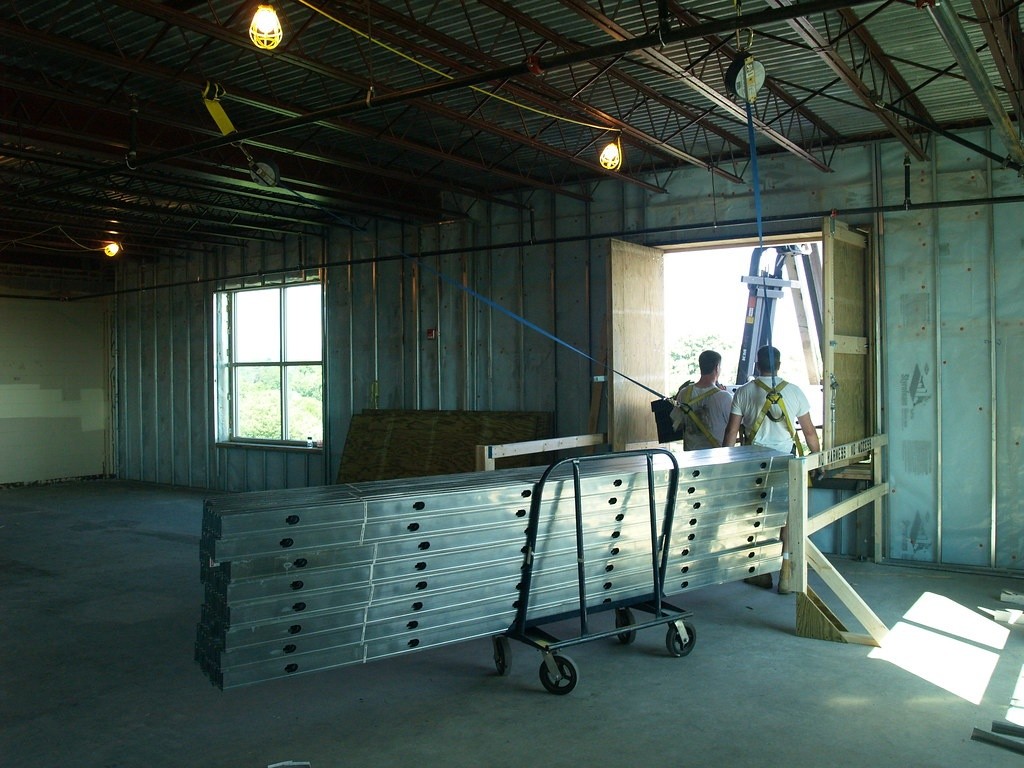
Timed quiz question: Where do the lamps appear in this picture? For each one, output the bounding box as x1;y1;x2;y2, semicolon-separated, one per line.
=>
249;0;283;49
600;129;623;171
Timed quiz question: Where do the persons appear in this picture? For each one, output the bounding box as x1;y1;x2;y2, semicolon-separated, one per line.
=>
670;350;731;456
721;346;821;589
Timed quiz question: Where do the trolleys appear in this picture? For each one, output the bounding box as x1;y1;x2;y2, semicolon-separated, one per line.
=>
492;447;698;694
